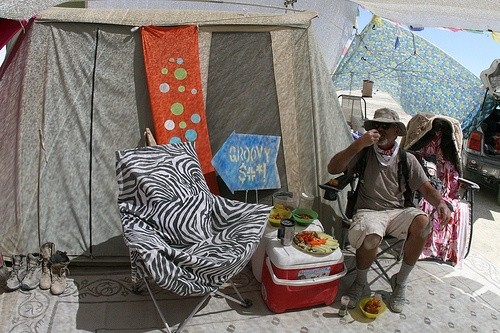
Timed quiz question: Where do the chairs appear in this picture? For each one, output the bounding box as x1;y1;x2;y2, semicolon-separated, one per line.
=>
114;140;274;333
319;149;455;286
338;95;369;129
406;118;479;258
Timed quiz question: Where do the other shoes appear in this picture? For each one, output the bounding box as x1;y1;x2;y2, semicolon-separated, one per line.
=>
390;272;407;313
346;281;366;309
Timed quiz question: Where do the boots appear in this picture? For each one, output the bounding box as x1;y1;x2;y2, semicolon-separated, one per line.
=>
41;242;56;290
21;252;43;289
49;250;69;295
6;253;29;290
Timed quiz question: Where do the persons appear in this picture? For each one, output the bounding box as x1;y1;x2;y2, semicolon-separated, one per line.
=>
327;108;451;314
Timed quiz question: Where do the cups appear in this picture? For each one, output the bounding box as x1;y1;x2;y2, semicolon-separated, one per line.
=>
277;219;296;246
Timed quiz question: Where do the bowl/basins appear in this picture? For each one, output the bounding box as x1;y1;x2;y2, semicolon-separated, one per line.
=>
292;208;318;227
359;297;386;319
268;209;292;227
273;192;297;209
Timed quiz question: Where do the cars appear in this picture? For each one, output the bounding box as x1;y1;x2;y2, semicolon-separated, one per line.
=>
460;58;500;193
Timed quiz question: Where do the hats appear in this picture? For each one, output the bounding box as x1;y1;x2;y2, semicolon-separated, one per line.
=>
364;108;407;136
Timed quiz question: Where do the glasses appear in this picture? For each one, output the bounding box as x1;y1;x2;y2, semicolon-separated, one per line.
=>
372;121;395;129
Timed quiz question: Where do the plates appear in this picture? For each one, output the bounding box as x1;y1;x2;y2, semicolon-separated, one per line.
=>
293;230;339;254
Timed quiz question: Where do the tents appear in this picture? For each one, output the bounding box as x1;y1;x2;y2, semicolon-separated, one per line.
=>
0;8;355;267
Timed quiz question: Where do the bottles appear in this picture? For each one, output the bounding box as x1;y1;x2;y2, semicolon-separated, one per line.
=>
298;192;315;210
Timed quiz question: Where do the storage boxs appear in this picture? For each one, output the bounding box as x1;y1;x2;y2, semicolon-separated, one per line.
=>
261;238;348;313
251;217;325;283
